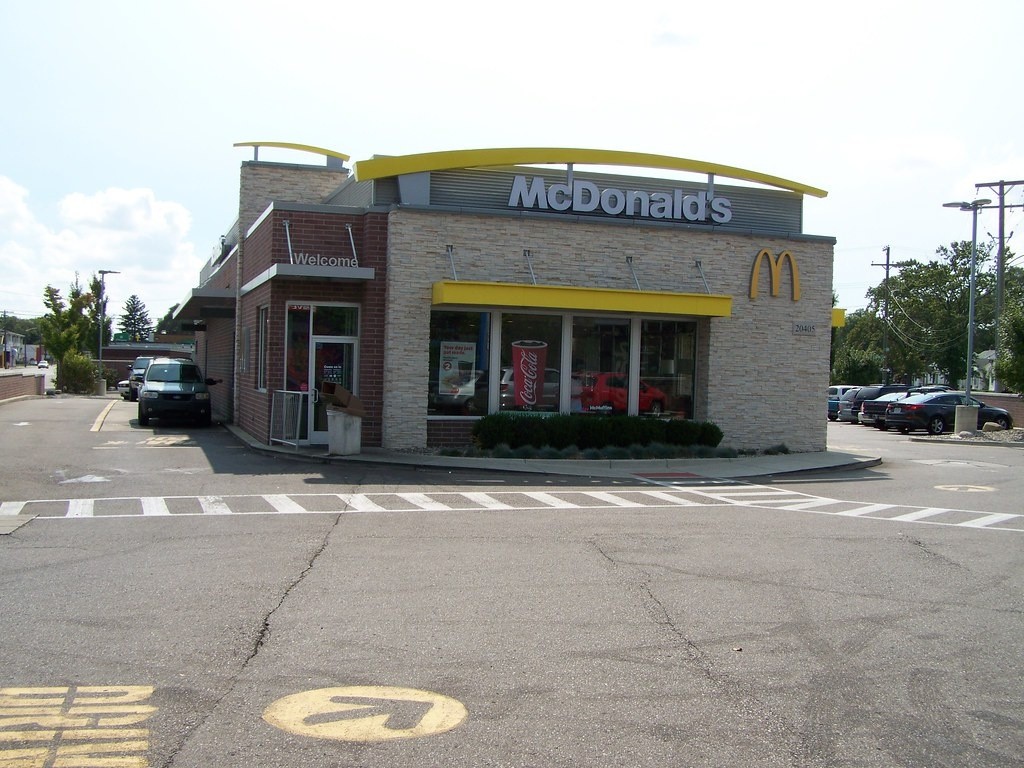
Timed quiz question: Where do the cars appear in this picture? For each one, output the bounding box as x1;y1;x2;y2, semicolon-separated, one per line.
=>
38;361;49;370
435;368;486;413
117;378;130;400
828;385;965;431
884;392;1015;435
473;367;583;411
584;371;668;417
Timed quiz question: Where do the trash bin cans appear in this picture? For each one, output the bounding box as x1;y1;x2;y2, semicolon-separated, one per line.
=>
325;409;362;455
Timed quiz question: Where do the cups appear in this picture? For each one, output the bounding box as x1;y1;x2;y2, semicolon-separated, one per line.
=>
512;339;548;406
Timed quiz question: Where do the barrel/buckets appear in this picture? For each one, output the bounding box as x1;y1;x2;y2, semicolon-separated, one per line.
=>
325;410;361;455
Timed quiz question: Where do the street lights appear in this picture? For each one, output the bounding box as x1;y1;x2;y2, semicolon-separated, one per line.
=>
941;198;992;436
94;270;121;395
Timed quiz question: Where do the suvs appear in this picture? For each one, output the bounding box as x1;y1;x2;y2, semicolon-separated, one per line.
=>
127;355;162;401
136;356;215;427
